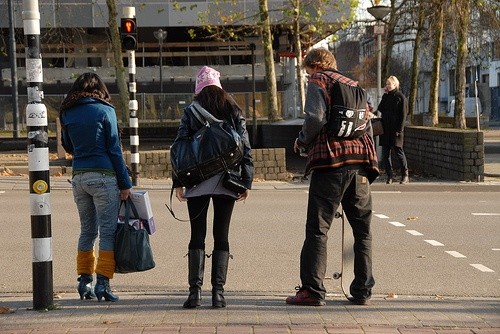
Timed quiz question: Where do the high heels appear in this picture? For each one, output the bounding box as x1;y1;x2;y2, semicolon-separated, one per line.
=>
78;274;96;300
95;275;119;301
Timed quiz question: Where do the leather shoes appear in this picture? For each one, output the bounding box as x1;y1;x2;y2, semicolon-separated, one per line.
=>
387;178;393;184
401;175;409;184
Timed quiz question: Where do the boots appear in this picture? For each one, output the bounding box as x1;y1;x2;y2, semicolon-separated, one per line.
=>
212;250;229;308
183;249;205;307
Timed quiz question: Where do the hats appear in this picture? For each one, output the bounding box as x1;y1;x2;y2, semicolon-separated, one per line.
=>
195;66;222;95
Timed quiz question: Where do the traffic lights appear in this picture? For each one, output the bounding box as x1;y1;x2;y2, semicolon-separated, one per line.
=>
120;17;138;51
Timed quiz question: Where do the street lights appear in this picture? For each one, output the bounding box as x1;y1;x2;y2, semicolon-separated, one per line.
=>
368;6;394;108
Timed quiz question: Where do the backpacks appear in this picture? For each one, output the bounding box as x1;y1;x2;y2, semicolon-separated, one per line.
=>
311;71;367;139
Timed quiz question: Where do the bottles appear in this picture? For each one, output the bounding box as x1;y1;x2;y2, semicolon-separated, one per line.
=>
299;147;308;157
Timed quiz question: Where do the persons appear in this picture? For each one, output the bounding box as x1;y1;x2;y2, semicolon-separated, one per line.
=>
59;72;132;301
176;66;253;307
374;75;408;183
286;47;379;305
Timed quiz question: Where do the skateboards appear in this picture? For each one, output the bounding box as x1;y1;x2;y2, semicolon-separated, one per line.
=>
334;207;357;302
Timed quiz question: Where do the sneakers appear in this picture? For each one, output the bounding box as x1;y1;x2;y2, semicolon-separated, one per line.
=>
356;299;369;304
286;286;326;305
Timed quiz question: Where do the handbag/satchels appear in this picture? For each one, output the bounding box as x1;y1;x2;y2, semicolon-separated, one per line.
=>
115;196;155;273
119;192;155;235
171;106;244;189
221;169;247;196
